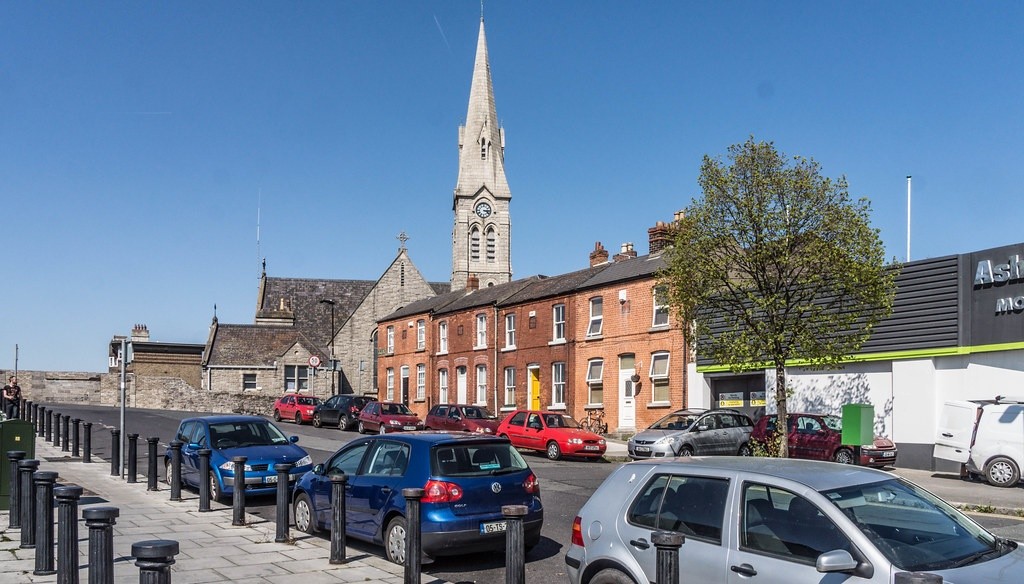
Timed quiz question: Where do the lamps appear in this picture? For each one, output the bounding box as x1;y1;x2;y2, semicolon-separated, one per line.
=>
631;361;643;382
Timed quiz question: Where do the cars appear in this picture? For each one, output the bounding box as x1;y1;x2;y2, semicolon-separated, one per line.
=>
164;411;315;502
273;393;323;425
496;410;607;462
293;429;545;568
312;394;378;432
564;456;1024;584
628;407;755;461
358;401;425;435
423;403;500;436
750;412;898;467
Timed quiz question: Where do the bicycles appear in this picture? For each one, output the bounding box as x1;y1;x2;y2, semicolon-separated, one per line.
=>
579;408;608;435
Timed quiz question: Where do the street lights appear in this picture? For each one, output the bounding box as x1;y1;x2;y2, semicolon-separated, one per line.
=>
320;298;336;396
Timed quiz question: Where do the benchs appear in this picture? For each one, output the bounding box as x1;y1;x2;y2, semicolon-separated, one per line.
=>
644;482;725;539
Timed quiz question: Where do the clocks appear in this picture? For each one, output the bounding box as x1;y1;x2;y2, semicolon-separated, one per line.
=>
476;202;491;218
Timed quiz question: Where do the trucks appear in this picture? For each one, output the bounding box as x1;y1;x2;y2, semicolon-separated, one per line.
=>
930;395;1024;488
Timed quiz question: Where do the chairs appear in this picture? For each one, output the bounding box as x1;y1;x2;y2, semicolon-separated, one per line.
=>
438;450;456;474
746;499;811;556
377;451;406;476
465;449;495;472
789;497;842;554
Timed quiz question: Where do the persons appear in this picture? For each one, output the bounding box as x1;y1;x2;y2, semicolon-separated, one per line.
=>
3;376;21;419
